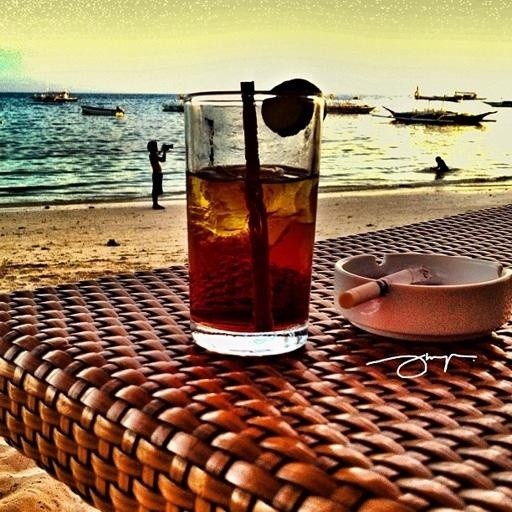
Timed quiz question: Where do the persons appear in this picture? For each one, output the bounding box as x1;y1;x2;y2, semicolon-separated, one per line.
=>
428;156;451;172
146;140;172;210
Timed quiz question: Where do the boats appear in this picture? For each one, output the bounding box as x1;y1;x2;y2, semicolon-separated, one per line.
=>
326;98;375;114
414;85;512;108
381;106;497;124
30;91;78;103
162;104;184;113
80;105;126;117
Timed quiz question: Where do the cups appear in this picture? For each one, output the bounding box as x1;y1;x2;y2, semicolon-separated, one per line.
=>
184;89;327;359
